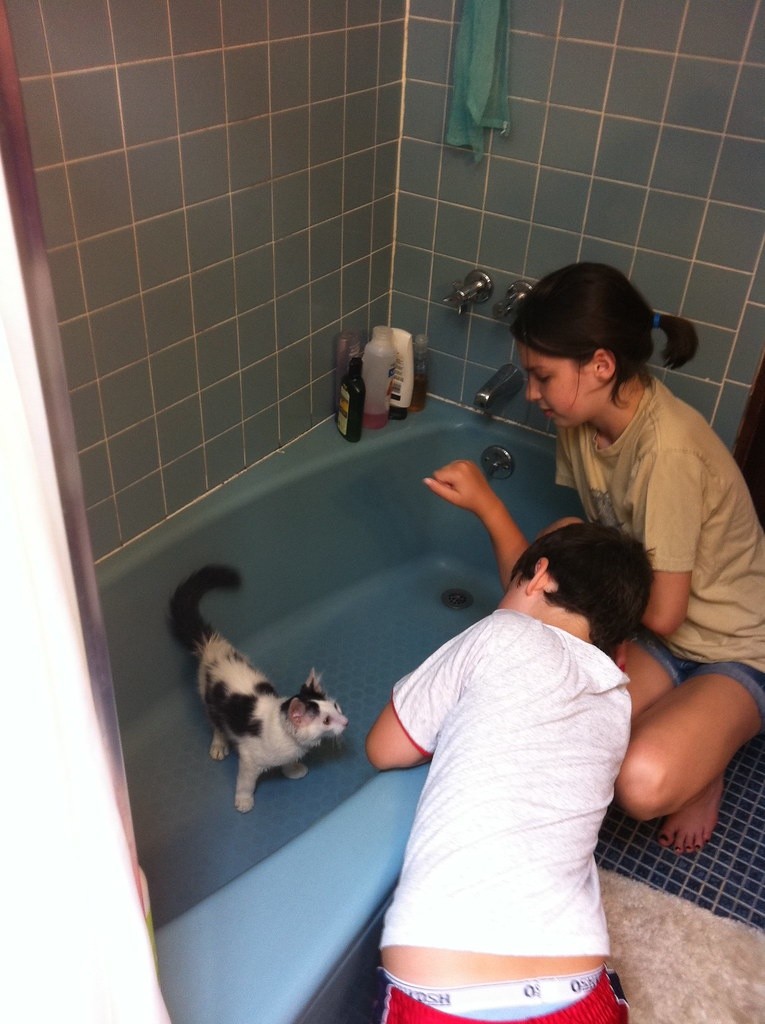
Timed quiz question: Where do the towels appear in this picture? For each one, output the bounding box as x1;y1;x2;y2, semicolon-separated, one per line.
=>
444;1;513;165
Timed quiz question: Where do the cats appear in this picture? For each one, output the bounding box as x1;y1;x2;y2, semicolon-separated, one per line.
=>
166;561;349;815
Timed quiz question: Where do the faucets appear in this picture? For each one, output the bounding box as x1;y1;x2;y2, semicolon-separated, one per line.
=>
473;364;524;408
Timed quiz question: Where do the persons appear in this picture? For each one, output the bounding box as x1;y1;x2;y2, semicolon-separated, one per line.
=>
368;461;655;1024
510;263;765;853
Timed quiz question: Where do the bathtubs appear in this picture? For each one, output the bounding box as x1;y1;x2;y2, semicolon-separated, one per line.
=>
91;391;593;1023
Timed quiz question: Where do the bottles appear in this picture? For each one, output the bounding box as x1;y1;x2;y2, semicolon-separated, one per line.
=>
334;325;428;443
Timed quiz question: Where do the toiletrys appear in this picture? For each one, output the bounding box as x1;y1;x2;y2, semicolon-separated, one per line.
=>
335;324;430;443
